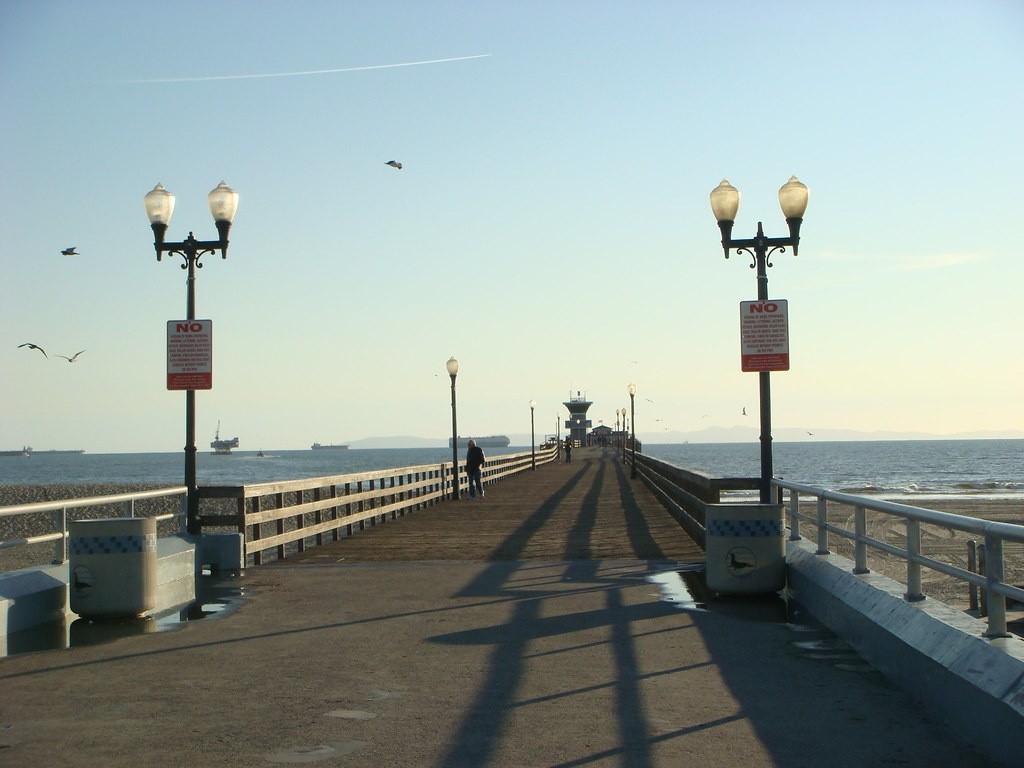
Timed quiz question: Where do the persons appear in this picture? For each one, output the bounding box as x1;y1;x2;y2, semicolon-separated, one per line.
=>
466;440;486;500
565;440;573;464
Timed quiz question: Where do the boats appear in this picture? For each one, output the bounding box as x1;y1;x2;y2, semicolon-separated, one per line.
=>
311;442;351;450
257;451;264;458
449;435;510;447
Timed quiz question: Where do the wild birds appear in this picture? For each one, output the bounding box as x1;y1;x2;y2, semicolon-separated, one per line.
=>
742;407;747;416
56;350;87;362
18;343;47;358
61;247;80;256
384;160;403;169
807;432;814;435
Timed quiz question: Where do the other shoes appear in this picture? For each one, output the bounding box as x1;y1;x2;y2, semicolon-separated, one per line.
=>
479;490;484;499
466;495;475;500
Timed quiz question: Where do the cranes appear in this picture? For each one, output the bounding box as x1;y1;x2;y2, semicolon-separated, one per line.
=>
215;420;221;441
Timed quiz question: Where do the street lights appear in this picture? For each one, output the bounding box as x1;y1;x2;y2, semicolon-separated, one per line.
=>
617;410;619;450
628;383;637;479
710;176;808;503
446;357;461;499
557;412;561;459
576;419;580;448
529;400;536;470
145;181;239;534
622;408;626;464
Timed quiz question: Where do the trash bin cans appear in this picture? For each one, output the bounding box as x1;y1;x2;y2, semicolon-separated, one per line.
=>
705;502;788;600
66;518;156;621
